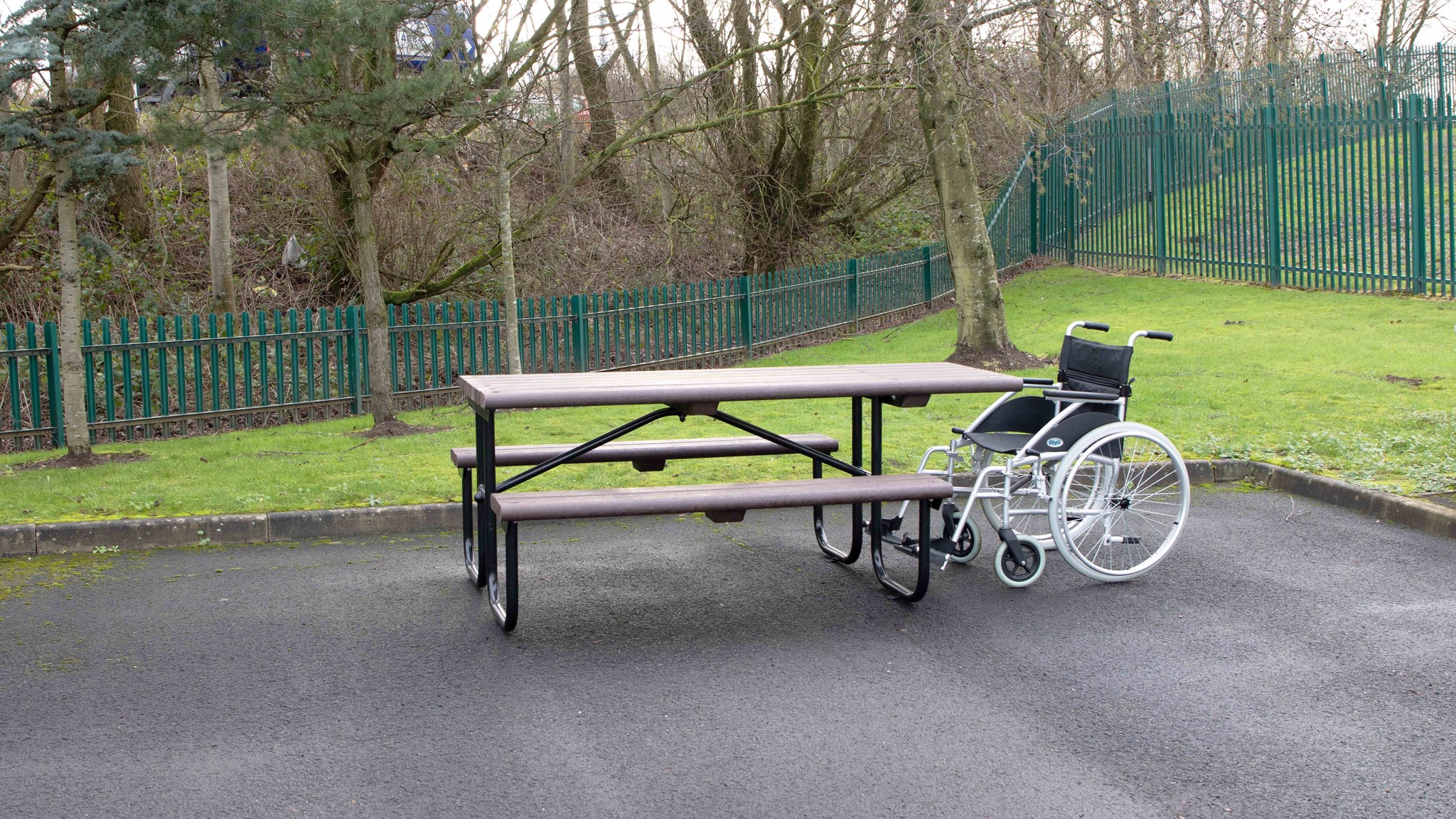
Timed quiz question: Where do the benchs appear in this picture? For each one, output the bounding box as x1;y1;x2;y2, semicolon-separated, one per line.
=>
451;434;839;586
483;474;953;630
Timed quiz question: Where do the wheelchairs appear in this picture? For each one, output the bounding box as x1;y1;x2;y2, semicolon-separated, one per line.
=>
865;320;1191;589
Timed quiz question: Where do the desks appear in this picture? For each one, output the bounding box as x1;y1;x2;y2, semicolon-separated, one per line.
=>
458;361;1024;630
